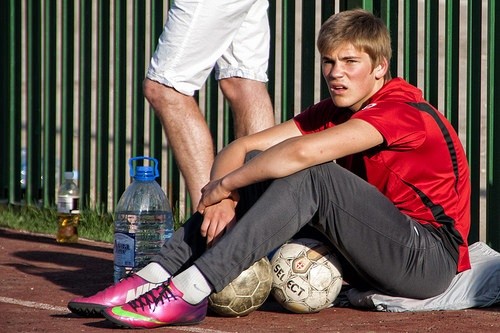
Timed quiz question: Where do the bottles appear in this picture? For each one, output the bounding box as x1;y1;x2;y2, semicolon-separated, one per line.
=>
57;172;80;245
113;158;175;286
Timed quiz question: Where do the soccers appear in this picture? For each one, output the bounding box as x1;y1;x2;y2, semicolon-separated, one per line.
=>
207;254;273;316
269;238;343;313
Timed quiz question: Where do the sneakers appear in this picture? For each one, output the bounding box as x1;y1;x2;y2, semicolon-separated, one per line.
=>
67;272;164;318
102;276;209;329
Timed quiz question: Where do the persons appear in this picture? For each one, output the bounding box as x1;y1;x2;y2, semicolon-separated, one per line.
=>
67;9;471;328
142;0;276;217
